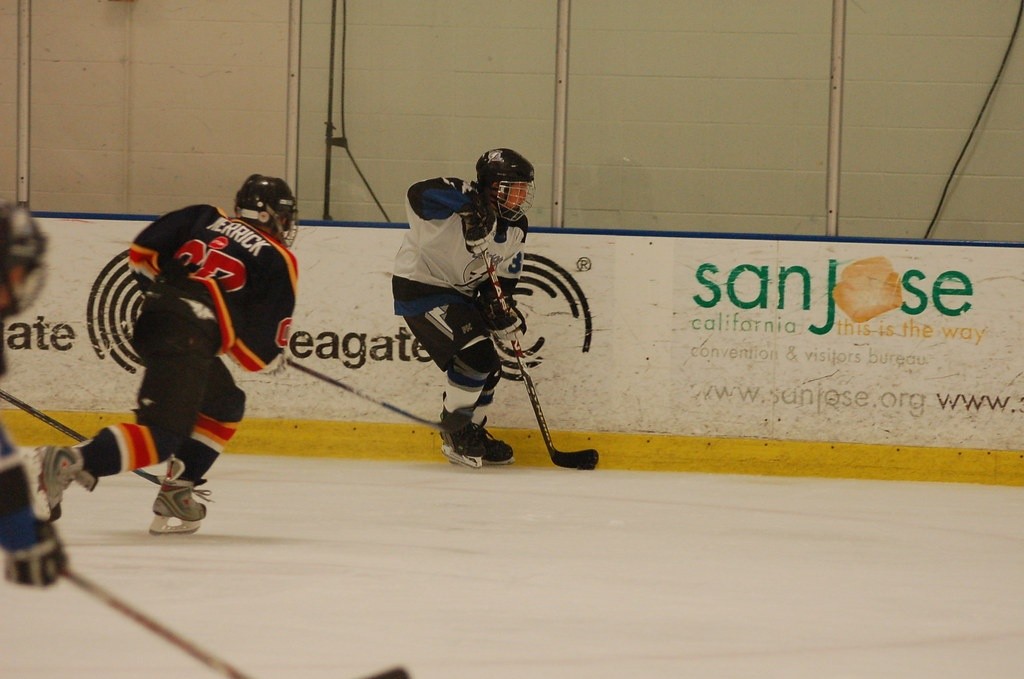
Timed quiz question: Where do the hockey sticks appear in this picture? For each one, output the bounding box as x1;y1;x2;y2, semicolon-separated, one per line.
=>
1;390;175;485
285;359;493;430
477;241;599;469
62;570;409;677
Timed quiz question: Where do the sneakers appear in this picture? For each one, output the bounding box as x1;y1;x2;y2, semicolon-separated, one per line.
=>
149;452;215;537
439;392;515;470
26;439;98;523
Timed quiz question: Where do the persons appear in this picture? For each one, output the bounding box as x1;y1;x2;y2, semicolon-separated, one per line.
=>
0;202;69;589
392;149;536;469
16;174;299;536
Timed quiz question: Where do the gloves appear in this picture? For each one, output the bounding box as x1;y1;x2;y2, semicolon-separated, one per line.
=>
472;287;526;340
458;181;497;254
5;520;68;586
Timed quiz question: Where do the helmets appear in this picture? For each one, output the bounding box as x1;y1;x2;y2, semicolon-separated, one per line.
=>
476;148;535;195
234;174;296;228
0;201;42;268
